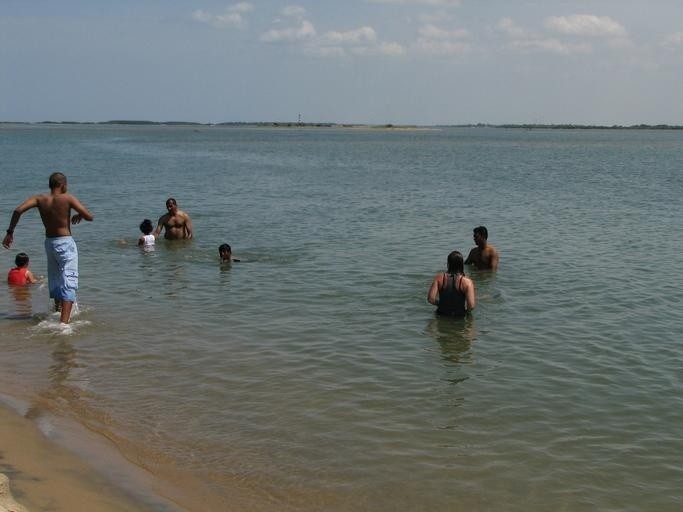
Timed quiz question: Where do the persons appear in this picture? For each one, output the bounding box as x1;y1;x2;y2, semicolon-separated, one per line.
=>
136;218;156;244
217;243;241;262
426;250;476;317
6;252;45;286
1;171;94;325
429;317;473;373
462;224;499;274
153;198;193;240
